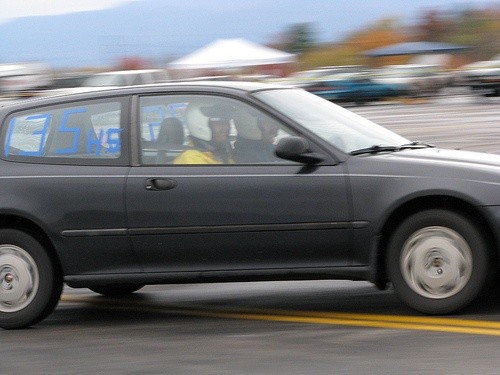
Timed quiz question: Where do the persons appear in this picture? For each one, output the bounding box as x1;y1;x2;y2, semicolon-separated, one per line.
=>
230;105;281;164
170;96;236;164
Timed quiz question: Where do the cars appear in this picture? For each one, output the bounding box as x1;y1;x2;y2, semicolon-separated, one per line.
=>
286;57;500;106
0;81;500;329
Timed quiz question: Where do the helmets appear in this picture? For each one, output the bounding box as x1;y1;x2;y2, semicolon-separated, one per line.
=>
233;103;281;141
183;97;237;142
